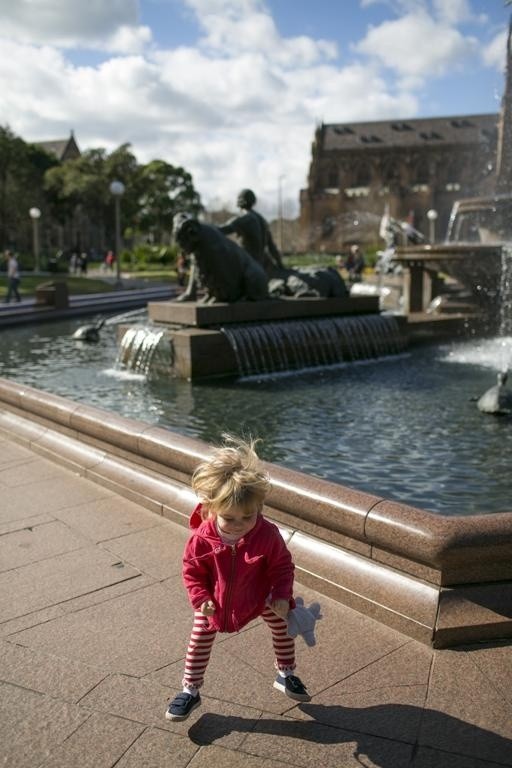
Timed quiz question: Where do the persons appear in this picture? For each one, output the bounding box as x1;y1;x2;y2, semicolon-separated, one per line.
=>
172;247;187;283
159;432;321;721
4;249;23;303
345;245;365;284
335;257;345;270
78;253;89;276
105;249;114;273
213;188;286;268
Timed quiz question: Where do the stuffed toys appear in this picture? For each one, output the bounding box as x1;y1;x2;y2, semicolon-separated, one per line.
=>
284;596;323;647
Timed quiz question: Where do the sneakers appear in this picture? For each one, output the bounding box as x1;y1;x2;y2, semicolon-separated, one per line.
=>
165;691;201;722
273;673;312;703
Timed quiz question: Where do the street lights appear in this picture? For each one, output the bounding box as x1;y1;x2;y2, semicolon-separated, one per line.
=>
277;173;285;249
28;206;43;273
428;208;438;244
111;180;129;282
